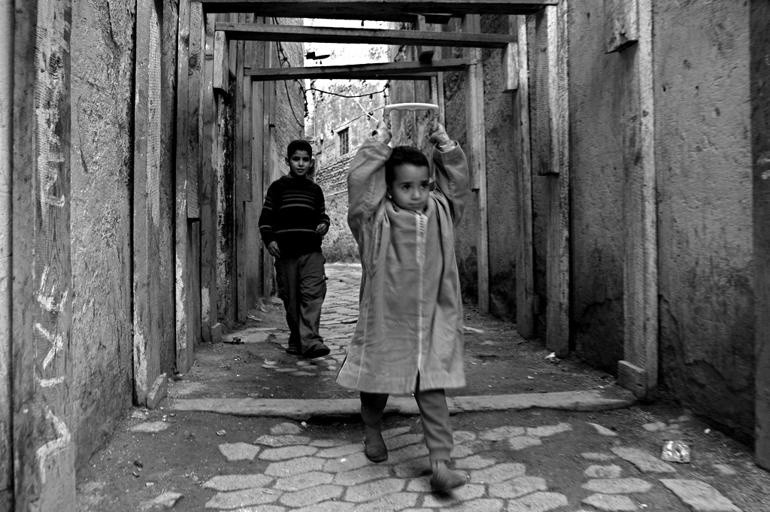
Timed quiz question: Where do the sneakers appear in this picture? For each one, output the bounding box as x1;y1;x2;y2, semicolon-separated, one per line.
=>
430;470;467;490
308;344;330;358
288;344;301;354
364;434;387;462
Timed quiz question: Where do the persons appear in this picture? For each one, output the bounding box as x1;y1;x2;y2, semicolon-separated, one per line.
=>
347;121;471;493
258;139;330;357
